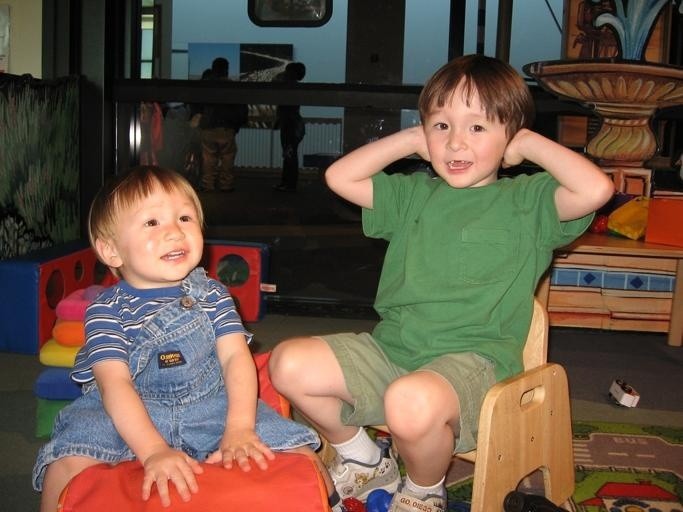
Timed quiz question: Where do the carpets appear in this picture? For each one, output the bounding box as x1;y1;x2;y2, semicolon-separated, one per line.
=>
298;394;683;512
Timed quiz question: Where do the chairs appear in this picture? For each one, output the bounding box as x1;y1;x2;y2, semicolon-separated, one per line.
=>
372;287;574;512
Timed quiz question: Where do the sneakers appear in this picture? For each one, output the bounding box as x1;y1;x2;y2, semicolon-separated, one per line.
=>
387;479;450;512
325;437;405;505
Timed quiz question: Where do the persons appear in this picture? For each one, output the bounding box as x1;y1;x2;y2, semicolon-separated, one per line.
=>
31;164;341;512
267;54;615;511
201;58;249;187
272;62;306;186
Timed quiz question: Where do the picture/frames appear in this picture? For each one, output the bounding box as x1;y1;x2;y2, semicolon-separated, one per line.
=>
243;0;336;28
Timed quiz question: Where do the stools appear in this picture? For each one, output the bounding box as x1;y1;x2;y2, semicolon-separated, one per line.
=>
59;347;334;512
